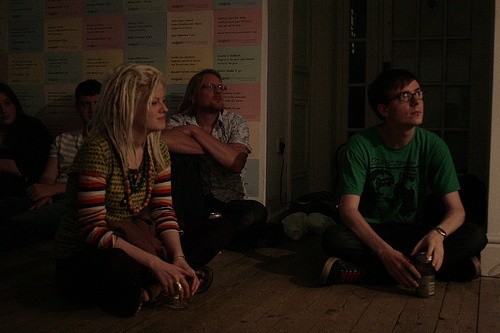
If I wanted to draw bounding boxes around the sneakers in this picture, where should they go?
[321,257,363,285]
[463,254,481,279]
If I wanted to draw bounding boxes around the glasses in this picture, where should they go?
[198,83,227,91]
[389,91,423,102]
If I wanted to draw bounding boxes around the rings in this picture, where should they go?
[174,281,183,291]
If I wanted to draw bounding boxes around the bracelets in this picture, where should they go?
[177,255,185,259]
[433,226,448,239]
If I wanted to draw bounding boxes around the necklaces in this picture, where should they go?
[103,125,154,212]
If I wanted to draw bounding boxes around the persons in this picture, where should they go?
[323,68,488,292]
[0,79,103,245]
[160,68,268,265]
[53,63,213,316]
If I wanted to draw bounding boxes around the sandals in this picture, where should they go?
[193,265,213,293]
[123,281,144,318]
